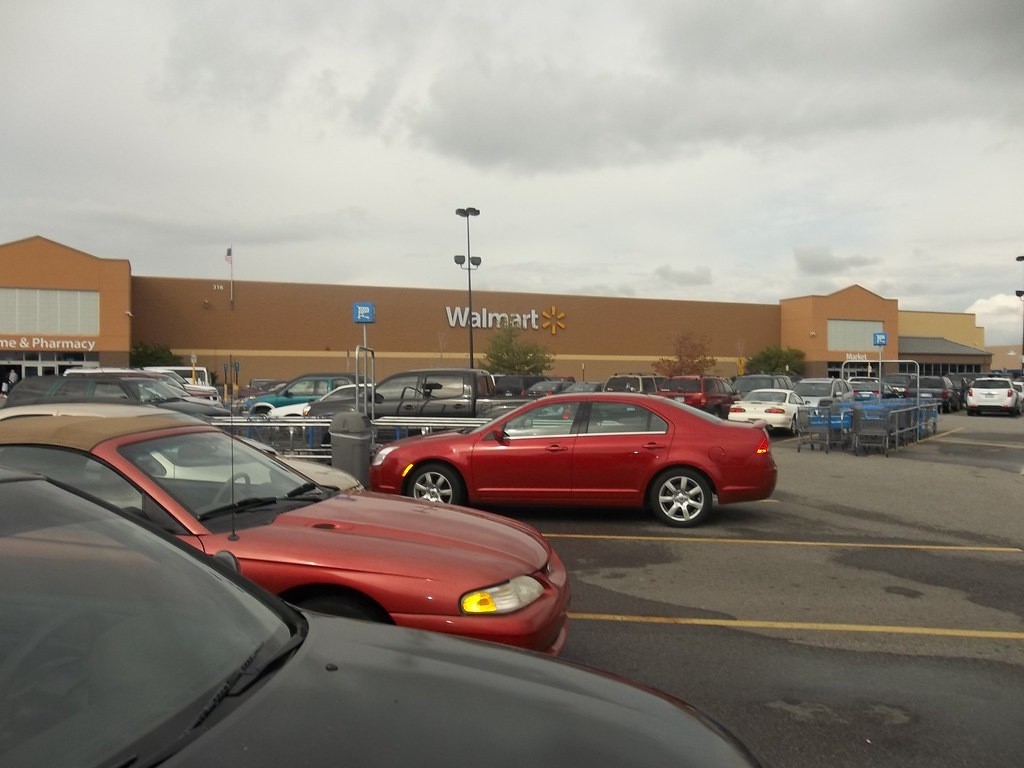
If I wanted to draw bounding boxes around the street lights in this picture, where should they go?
[1014,255,1024,382]
[454,208,481,367]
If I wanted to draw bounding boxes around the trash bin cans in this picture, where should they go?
[328,412,373,490]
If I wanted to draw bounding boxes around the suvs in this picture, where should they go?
[901,375,961,412]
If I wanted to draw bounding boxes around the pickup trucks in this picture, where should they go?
[304,369,581,463]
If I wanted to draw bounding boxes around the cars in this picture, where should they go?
[0,468,760,768]
[725,388,810,433]
[1010,382,1024,408]
[0,366,384,512]
[965,378,1022,416]
[489,371,921,420]
[0,403,574,664]
[367,391,777,528]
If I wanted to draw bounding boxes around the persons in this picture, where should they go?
[0,376,9,399]
[9,370,19,384]
